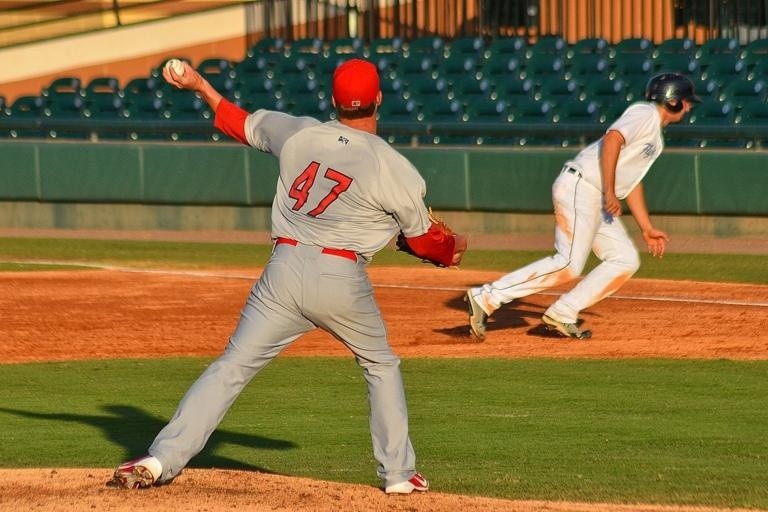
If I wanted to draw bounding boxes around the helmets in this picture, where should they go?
[646,72,702,112]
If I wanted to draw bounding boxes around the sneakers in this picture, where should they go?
[464,290,488,339]
[386,473,429,494]
[542,313,592,340]
[114,455,164,491]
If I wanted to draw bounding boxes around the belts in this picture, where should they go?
[563,166,582,178]
[275,238,357,260]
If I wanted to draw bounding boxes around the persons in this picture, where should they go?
[104,57,468,497]
[462,72,705,344]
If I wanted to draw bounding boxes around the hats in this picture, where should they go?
[333,60,380,109]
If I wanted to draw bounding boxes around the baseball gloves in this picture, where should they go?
[397,206,466,268]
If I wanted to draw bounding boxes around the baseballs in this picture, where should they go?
[164,59,186,78]
